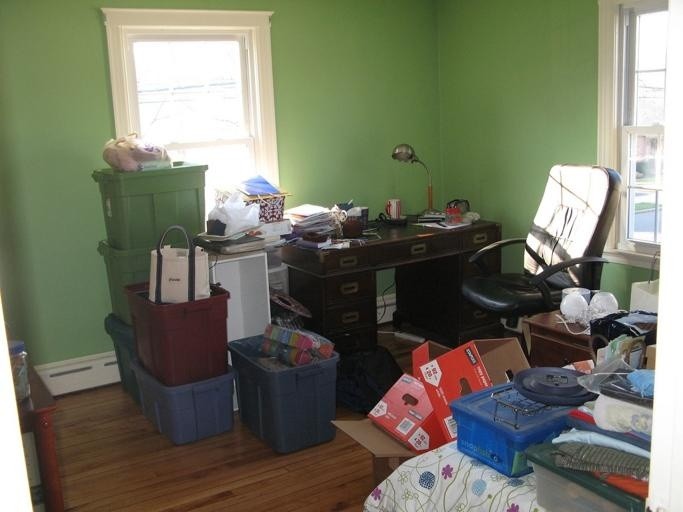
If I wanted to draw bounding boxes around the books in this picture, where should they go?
[193,203,351,255]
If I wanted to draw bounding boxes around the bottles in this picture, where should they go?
[7,342,31,403]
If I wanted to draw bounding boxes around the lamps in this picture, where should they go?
[393,144,444,218]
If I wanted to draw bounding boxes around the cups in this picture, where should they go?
[385,199,402,219]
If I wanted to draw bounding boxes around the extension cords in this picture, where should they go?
[395,331,425,344]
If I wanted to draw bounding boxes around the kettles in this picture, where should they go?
[445,199,471,225]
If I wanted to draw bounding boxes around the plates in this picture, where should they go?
[381,217,406,222]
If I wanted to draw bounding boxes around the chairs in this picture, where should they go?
[461,164,621,339]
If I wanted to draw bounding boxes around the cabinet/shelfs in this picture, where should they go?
[521,312,592,367]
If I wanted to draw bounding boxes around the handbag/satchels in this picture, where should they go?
[590,311,657,364]
[103,132,173,172]
[149,225,210,304]
[237,175,280,205]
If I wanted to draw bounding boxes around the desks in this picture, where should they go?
[19,357,64,512]
[279,218,503,408]
